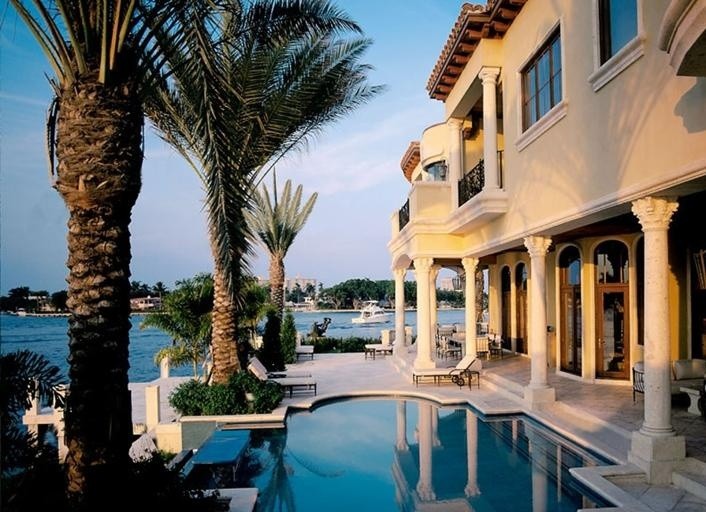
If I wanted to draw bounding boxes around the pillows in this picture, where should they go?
[693,357,705,380]
[673,358,696,384]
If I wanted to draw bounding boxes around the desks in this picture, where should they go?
[459,369,481,392]
[678,387,705,417]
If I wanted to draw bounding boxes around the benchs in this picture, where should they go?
[630,358,706,411]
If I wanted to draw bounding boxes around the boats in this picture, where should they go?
[349,296,391,325]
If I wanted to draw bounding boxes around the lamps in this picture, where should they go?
[436,157,448,179]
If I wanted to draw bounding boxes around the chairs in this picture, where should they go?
[411,353,478,389]
[244,342,316,399]
[362,320,506,364]
